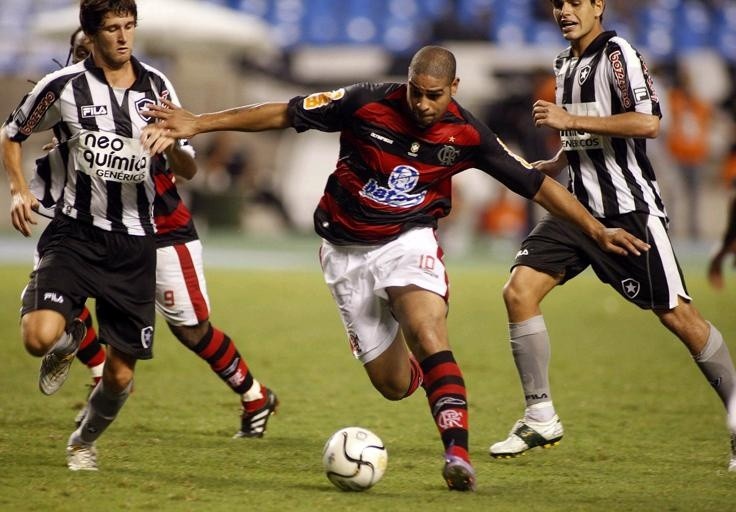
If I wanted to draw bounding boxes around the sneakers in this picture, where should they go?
[441,459,476,492]
[39,318,87,396]
[231,384,280,440]
[65,383,99,472]
[488,412,566,459]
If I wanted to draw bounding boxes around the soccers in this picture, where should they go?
[322,428,387,492]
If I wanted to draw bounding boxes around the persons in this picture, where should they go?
[23,25,281,444]
[140,45,656,490]
[0,0,198,470]
[488,1,736,470]
